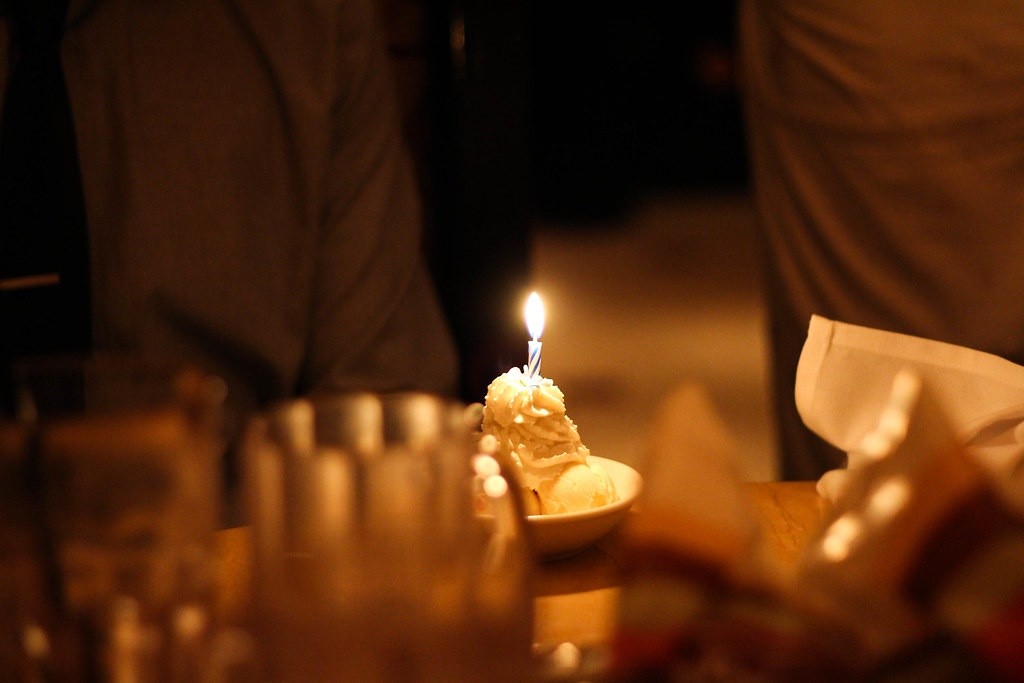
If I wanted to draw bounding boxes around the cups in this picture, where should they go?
[0,352,226,683]
[240,392,534,683]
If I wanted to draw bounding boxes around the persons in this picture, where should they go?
[0,0,463,502]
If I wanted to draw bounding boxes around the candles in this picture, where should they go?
[523,293,546,377]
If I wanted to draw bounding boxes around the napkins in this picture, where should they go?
[794,315,1024,521]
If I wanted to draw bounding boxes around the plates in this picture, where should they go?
[476,456,642,559]
